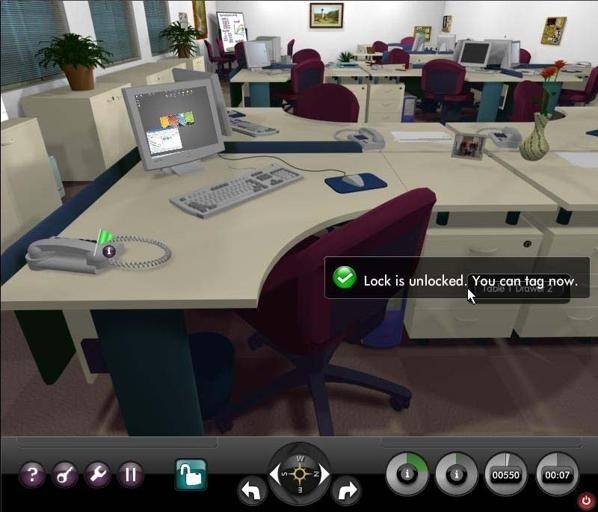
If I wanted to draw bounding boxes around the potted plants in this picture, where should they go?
[159,21,200,59]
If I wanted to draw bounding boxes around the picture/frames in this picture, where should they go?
[309,2,342,28]
